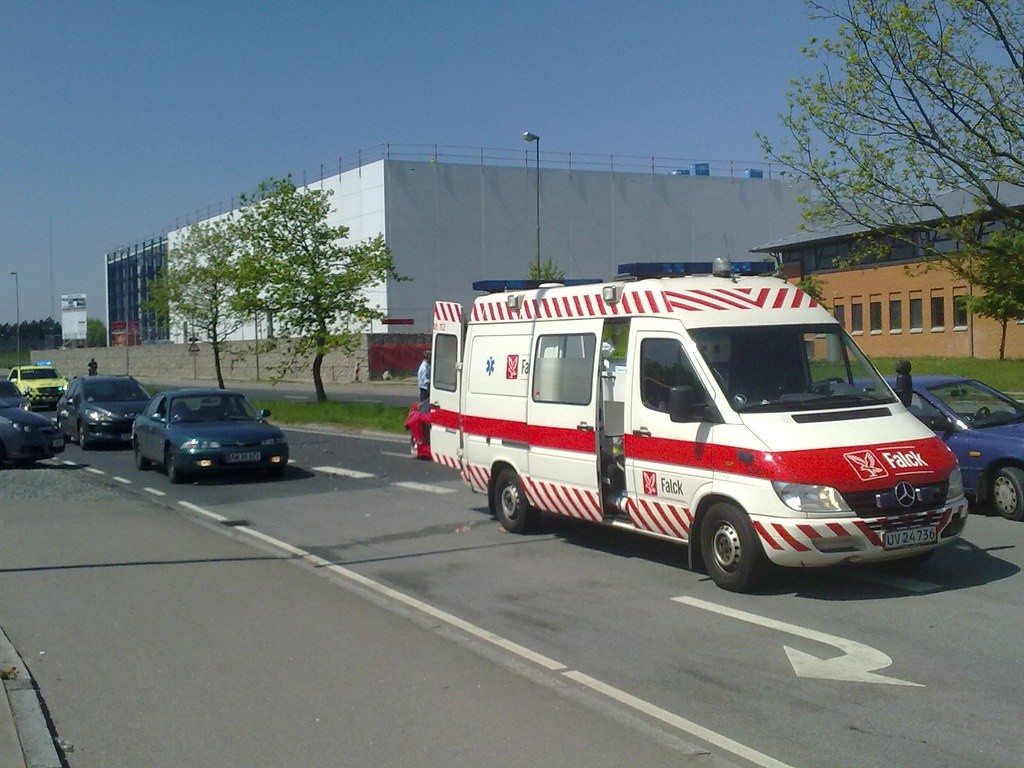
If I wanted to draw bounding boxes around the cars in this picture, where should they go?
[132,388,291,486]
[403,400,435,459]
[0,380,33,413]
[0,407,66,469]
[832,376,1024,525]
[7,365,71,410]
[56,375,158,453]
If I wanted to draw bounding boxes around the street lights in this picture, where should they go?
[523,131,545,291]
[9,272,21,366]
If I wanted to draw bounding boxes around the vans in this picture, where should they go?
[427,255,967,594]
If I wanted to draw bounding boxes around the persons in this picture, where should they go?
[215,395,241,420]
[87,357,99,376]
[416,348,431,402]
